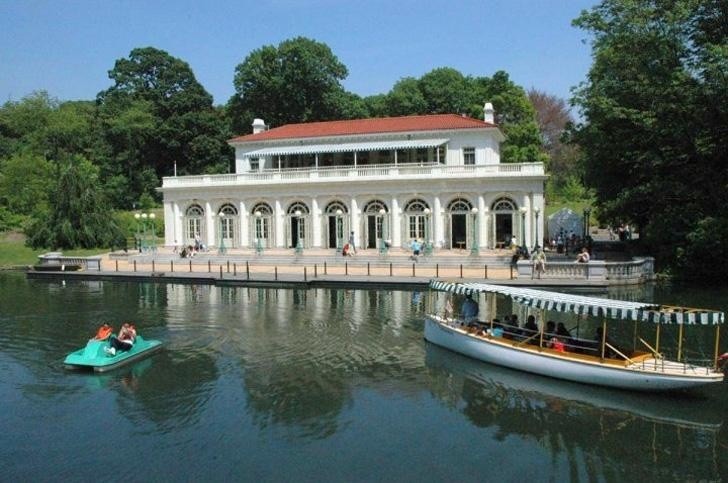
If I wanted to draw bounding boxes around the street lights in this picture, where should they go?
[254,210,264,257]
[218,212,227,255]
[470,208,479,258]
[134,213,158,256]
[294,210,303,256]
[518,206,528,254]
[335,209,344,257]
[422,207,433,257]
[582,207,591,236]
[531,206,540,255]
[378,208,388,257]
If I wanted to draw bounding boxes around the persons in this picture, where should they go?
[94,321,112,341]
[409,239,420,256]
[509,227,593,274]
[107,321,136,357]
[461,291,616,359]
[349,231,358,254]
[342,244,353,257]
[172,232,201,259]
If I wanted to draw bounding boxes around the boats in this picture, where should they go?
[62,333,163,372]
[423,278,727,390]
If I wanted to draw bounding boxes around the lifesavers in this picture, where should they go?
[717,353,728,368]
